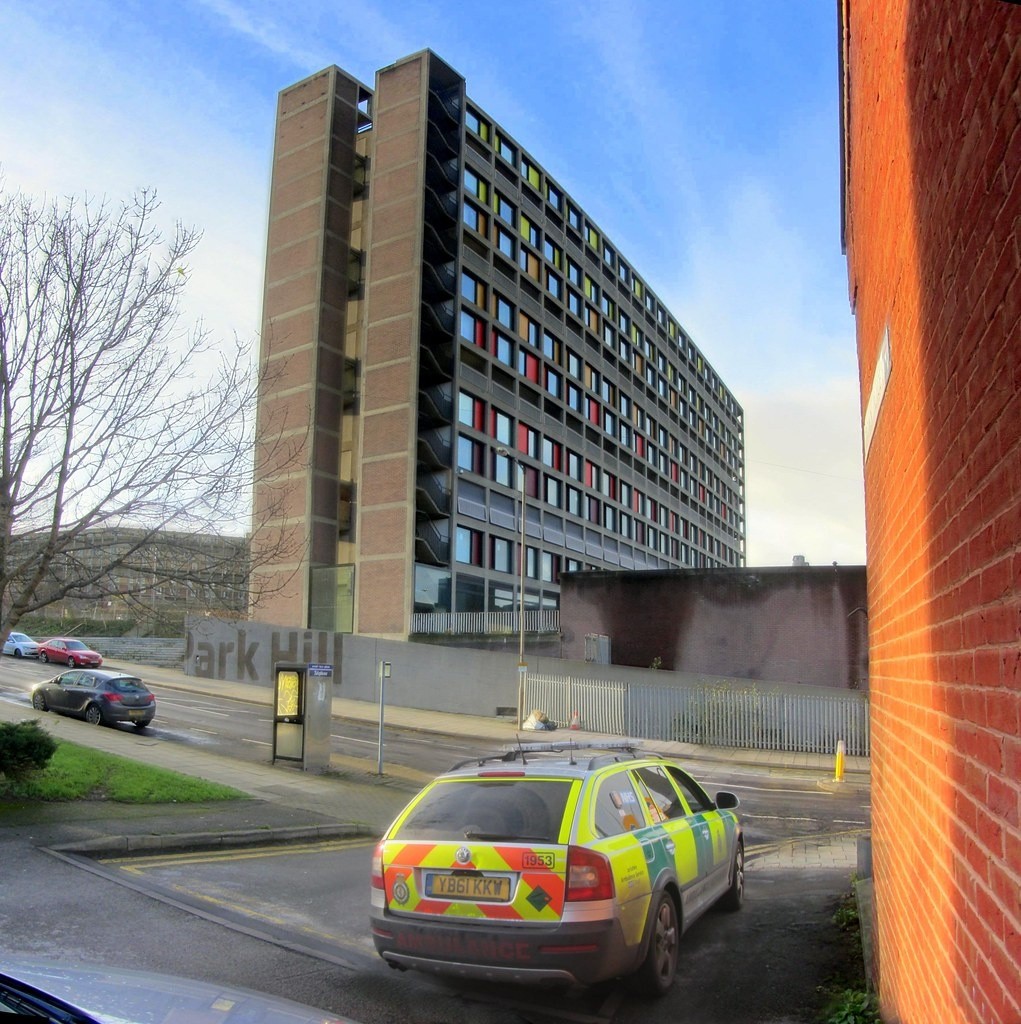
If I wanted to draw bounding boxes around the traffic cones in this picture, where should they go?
[571,710,581,731]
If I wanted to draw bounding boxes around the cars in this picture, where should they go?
[31,668,156,728]
[368,734,744,999]
[38,638,103,669]
[1,632,40,660]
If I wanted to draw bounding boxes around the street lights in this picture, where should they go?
[494,445,526,731]
